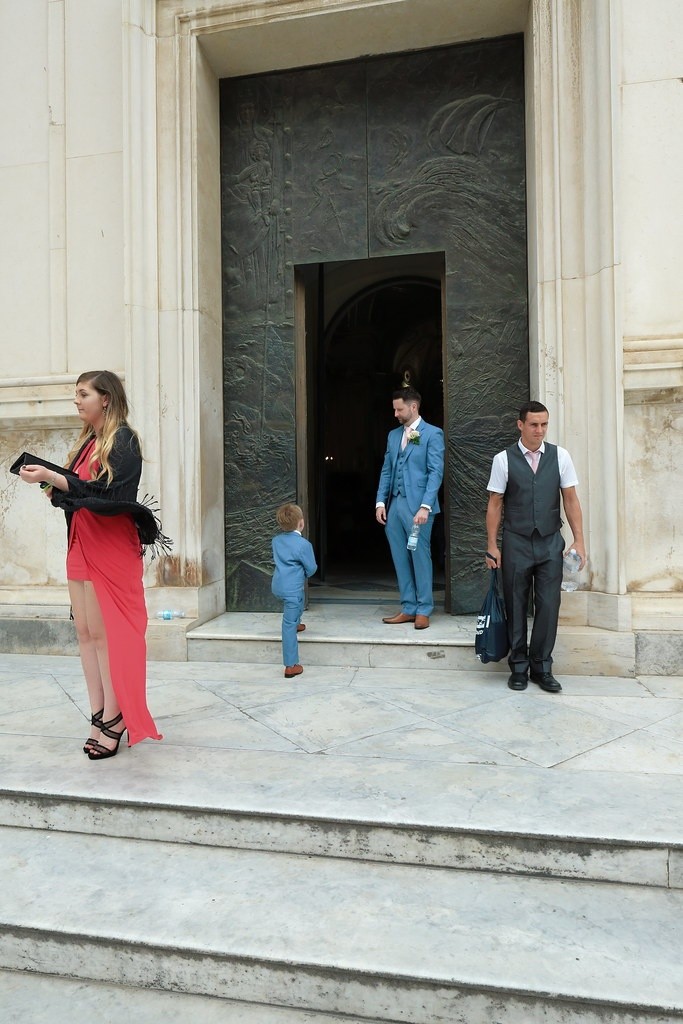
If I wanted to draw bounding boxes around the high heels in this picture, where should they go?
[88,711,131,760]
[84,707,105,754]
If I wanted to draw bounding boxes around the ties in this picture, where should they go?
[526,451,539,475]
[402,428,412,452]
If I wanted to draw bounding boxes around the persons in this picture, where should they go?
[486,400,586,691]
[18,370,174,761]
[271,504,318,678]
[375,388,445,629]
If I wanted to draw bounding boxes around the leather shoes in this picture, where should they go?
[414,615,430,629]
[297,624,306,632]
[507,672,530,690]
[529,668,562,691]
[284,664,304,678]
[382,611,416,625]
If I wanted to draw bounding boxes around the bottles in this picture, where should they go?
[155,610,185,620]
[561,549,582,593]
[406,522,420,551]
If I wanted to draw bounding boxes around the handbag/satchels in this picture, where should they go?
[474,551,511,664]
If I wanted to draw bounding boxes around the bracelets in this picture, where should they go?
[421,507,430,510]
[47,472,57,486]
